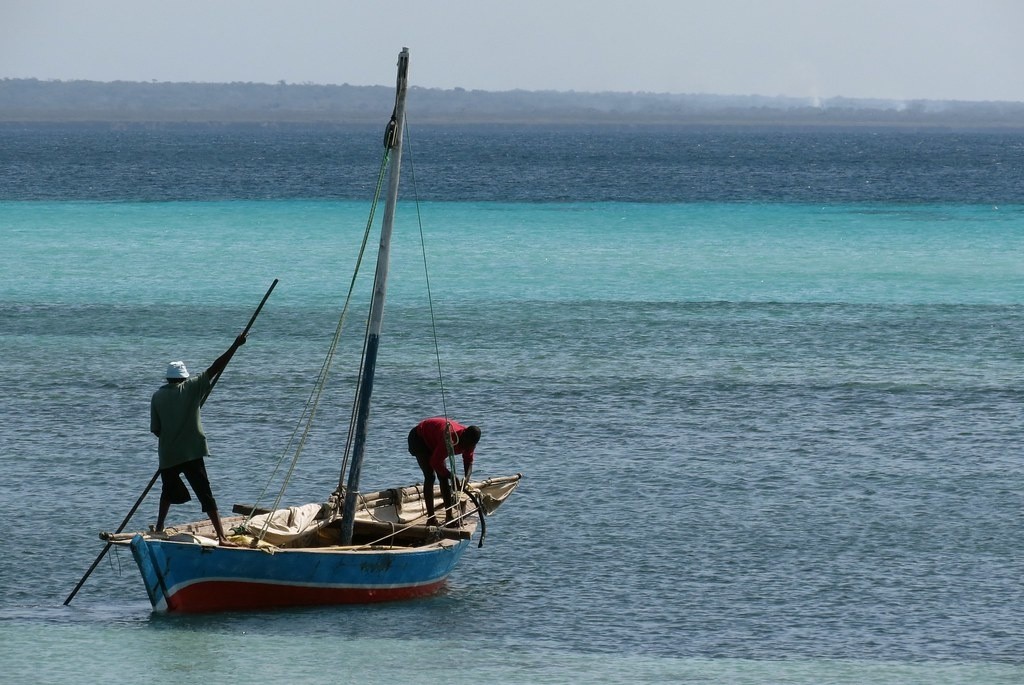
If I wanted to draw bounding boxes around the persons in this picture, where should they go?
[149,330,253,546]
[408,417,482,529]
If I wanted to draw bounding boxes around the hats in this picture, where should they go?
[165,360,189,378]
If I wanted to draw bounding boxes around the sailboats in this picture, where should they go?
[97,47,525,620]
